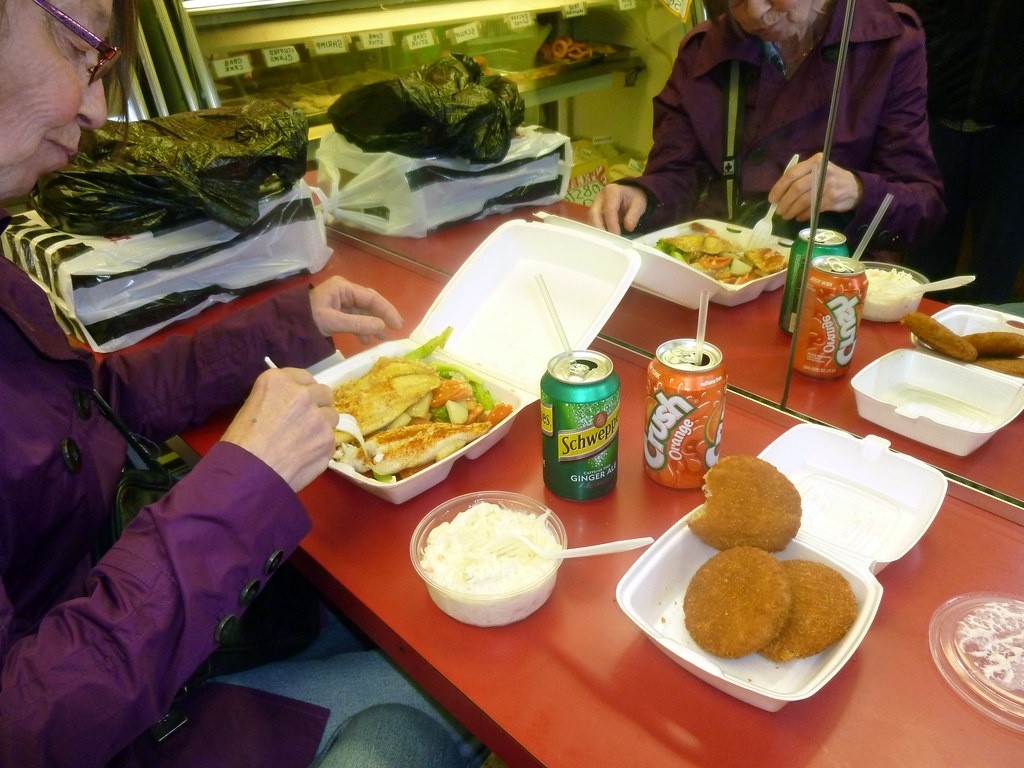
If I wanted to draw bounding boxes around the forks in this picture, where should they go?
[881,275,975,295]
[747,155,800,248]
[490,535,655,562]
[265,357,368,459]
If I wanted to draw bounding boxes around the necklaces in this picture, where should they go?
[782,34,824,69]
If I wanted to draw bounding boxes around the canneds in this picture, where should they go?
[642,338,727,490]
[540,349,620,501]
[791,256,869,380]
[778,227,850,335]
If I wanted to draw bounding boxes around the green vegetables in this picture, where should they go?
[401,324,493,423]
[653,239,690,263]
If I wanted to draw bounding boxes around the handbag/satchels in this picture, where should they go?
[112,463,318,679]
[0,179,331,353]
[328,52,527,165]
[26,95,310,236]
[308,117,573,239]
[732,196,812,240]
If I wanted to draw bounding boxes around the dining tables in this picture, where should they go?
[60,230,1023,768]
[294,169,1024,503]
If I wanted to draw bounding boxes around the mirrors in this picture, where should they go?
[122,1,1024,512]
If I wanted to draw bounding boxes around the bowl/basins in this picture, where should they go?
[410,491,568,627]
[860,261,929,322]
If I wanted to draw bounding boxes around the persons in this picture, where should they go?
[588,1,943,243]
[0,0,492,768]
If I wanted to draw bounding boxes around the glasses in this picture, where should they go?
[33,0,122,87]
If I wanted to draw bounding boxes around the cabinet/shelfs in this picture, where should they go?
[130,0,705,212]
